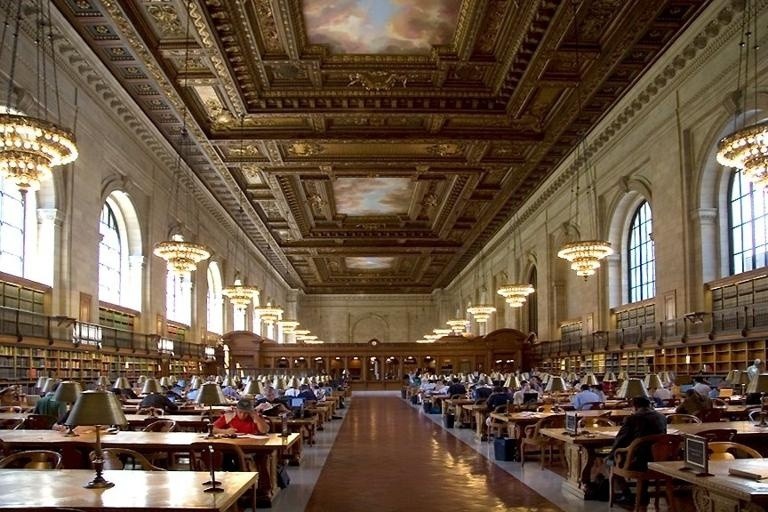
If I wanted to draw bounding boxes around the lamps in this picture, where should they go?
[254,170,285,328]
[417,370,768,427]
[294,254,323,345]
[219,114,259,315]
[277,225,300,334]
[557,3,613,280]
[152,1,210,274]
[715,3,767,189]
[0,0,80,199]
[465,176,497,324]
[497,113,535,309]
[35,372,333,489]
[413,210,471,344]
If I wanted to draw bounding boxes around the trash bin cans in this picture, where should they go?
[411,395,418,405]
[494,436,519,462]
[402,389,407,399]
[443,412,454,428]
[423,400,432,414]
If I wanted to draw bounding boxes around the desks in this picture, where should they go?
[0,384,350,512]
[400,383,768,512]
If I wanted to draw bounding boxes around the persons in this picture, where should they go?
[36,379,203,470]
[212,381,346,455]
[405,359,761,509]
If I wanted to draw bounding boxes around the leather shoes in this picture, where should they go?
[616,495,635,505]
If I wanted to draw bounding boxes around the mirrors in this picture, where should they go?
[275,356,475,382]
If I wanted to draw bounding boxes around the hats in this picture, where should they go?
[237,400,251,412]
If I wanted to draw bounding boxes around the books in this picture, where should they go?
[0,341,197,392]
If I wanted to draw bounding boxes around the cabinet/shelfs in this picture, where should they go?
[0,342,205,394]
[534,337,768,378]
